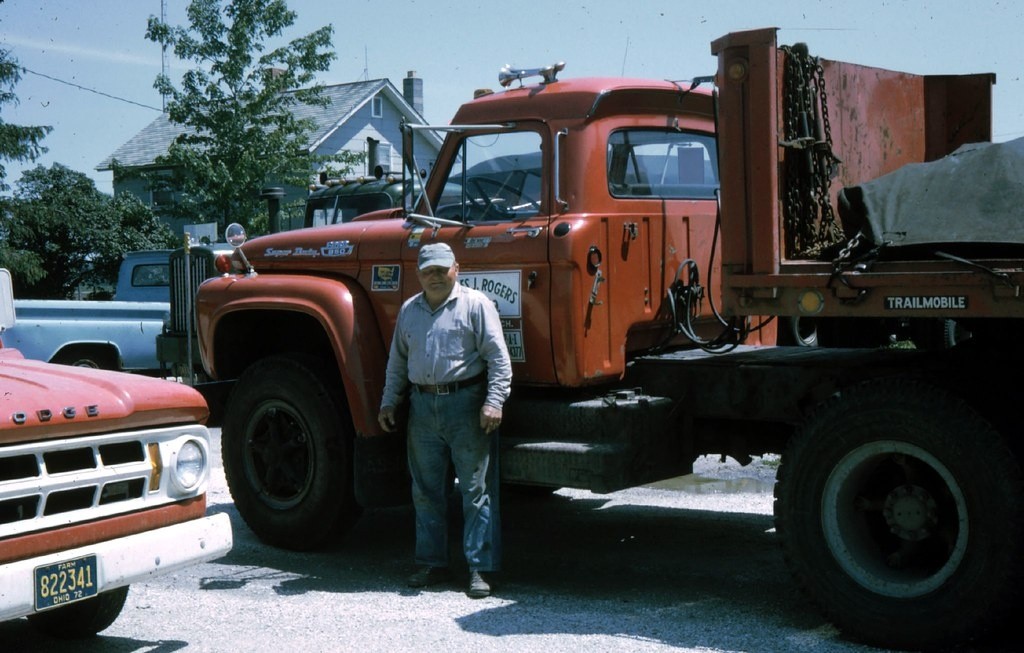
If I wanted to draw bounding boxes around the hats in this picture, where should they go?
[418,243,455,270]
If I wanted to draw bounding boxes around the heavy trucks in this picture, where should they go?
[195,29,1024,653]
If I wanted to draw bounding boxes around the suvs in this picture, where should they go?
[0,268,234,638]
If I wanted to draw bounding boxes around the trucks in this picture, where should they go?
[155,165,465,395]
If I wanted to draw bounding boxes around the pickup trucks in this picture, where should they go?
[1,250,180,377]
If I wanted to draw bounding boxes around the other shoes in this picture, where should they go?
[407,566,449,586]
[468,570,494,597]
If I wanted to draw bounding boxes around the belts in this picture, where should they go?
[411,373,484,396]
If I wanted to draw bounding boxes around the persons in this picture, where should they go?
[378,243,514,597]
